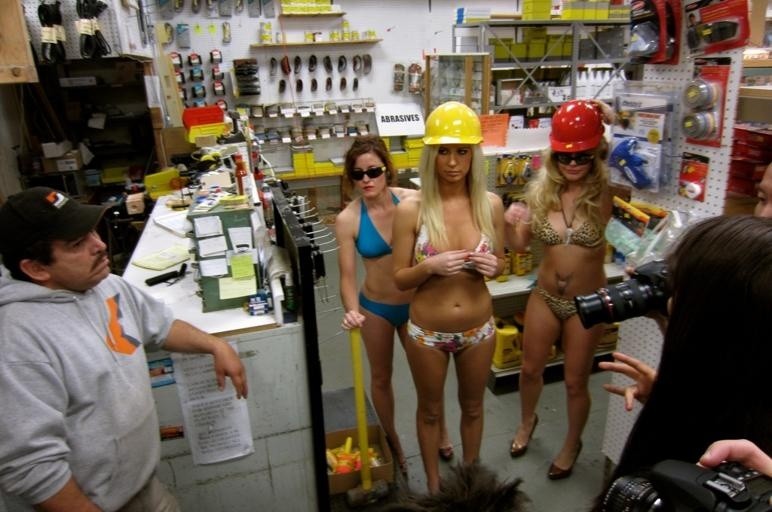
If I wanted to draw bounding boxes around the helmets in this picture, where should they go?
[422,101,484,145]
[550,99,605,153]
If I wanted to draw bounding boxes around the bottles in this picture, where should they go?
[306,19,376,42]
[114,192,127,205]
[235,159,273,221]
[563,69,626,100]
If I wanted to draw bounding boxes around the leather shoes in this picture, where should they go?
[549,440,582,479]
[511,413,538,456]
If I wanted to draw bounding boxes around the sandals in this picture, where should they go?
[438,441,454,460]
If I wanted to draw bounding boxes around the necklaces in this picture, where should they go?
[557,191,581,246]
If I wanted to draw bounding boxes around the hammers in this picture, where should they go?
[346,317,389,508]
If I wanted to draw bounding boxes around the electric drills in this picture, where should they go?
[606,137,653,190]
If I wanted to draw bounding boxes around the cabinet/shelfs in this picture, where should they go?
[0,0,169,206]
[482,128,625,396]
[452,20,633,127]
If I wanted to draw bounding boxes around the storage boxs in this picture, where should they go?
[325,424,398,494]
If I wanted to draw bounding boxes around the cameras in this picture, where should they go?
[574,259,676,330]
[601,459,772,512]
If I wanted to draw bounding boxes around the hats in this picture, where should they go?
[0,186,109,254]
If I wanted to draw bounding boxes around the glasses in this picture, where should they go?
[555,152,596,165]
[350,165,387,180]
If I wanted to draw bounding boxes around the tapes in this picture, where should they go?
[680,80,719,141]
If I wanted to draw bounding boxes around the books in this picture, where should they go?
[151,209,193,236]
[132,246,190,271]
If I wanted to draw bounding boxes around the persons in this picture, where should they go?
[696,438,771,478]
[0,188,248,512]
[502,93,620,481]
[335,456,534,511]
[586,214,770,512]
[335,133,454,479]
[598,160,772,412]
[392,101,506,497]
